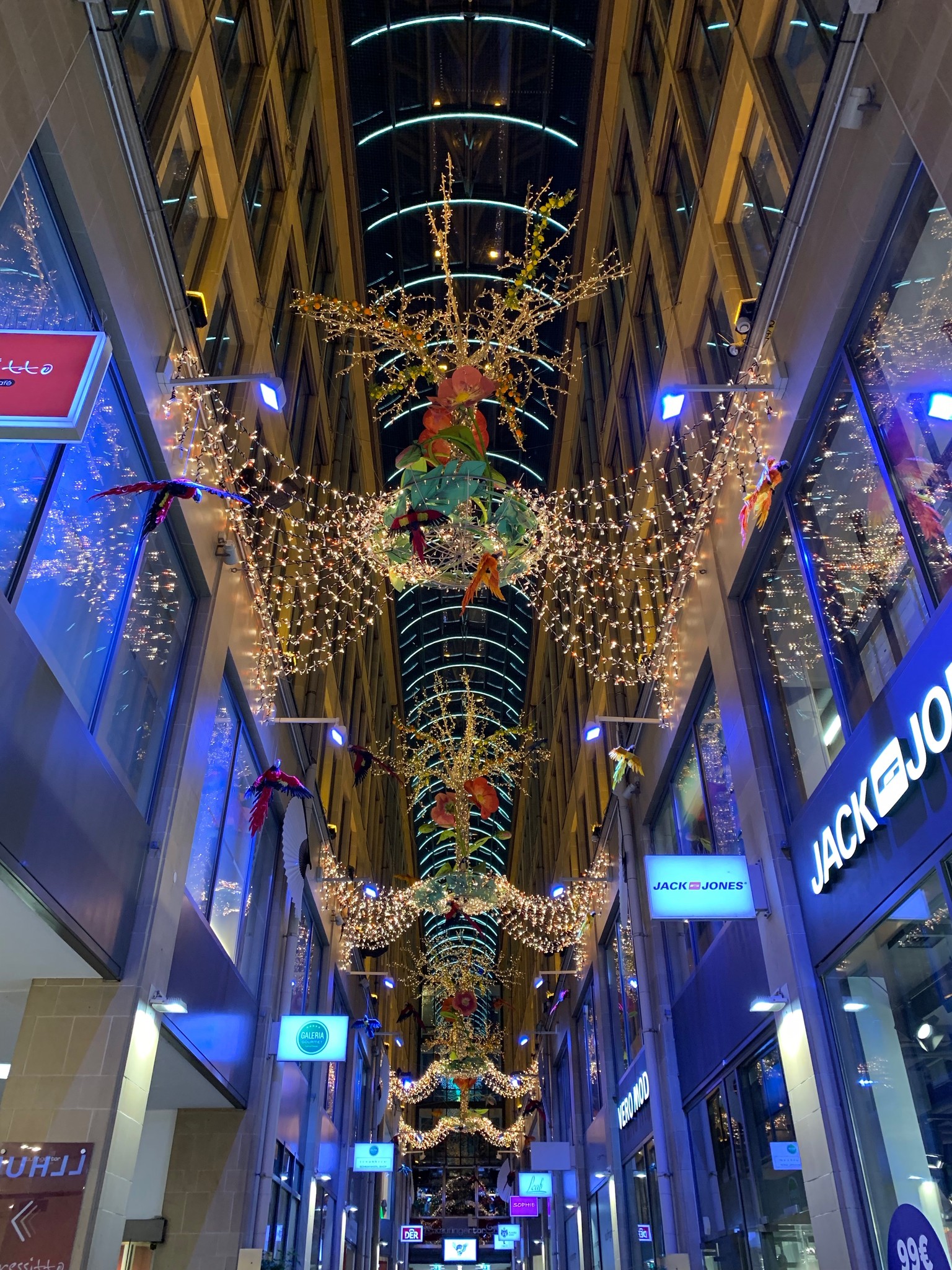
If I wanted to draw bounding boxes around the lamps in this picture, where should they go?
[373,1032,405,1048]
[659,360,790,420]
[519,1023,558,1046]
[315,866,378,898]
[534,971,577,989]
[156,355,287,414]
[551,866,613,898]
[345,962,395,984]
[584,703,669,741]
[266,702,348,747]
[509,1072,528,1088]
